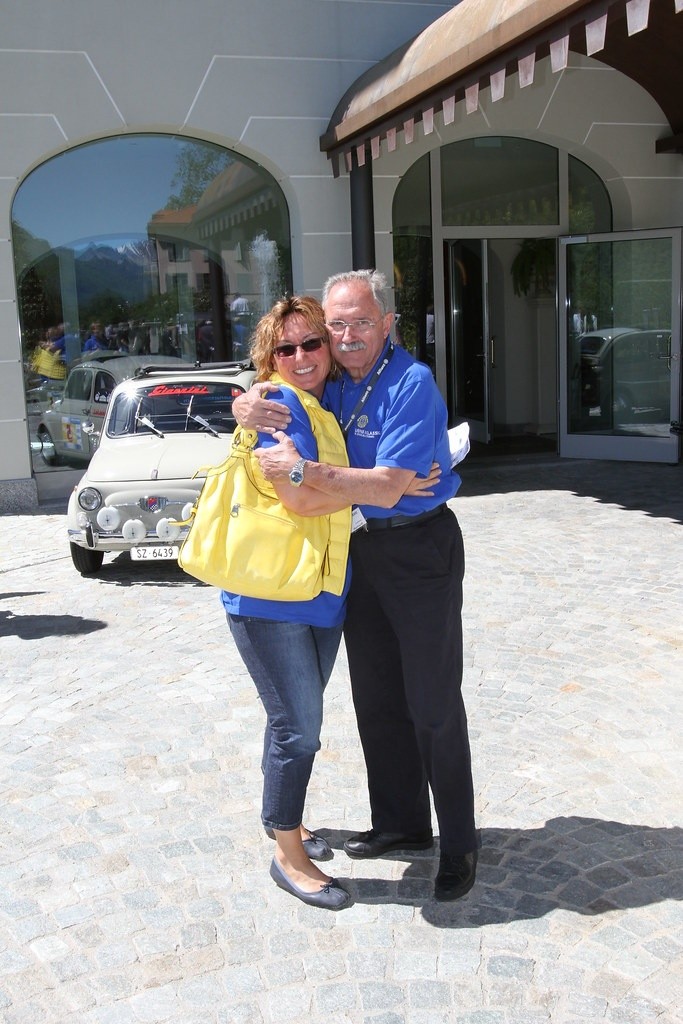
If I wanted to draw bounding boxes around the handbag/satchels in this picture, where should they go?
[176,388,329,602]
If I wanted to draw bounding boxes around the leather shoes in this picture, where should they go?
[342,828,435,860]
[433,849,479,901]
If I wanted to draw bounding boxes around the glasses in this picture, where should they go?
[323,315,386,336]
[272,335,330,358]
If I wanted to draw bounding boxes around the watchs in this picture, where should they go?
[289,458,308,487]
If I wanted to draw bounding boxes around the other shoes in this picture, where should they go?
[269,856,351,909]
[263,822,331,860]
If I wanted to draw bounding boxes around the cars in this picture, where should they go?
[576,326,673,424]
[67,358,257,574]
[37,355,188,467]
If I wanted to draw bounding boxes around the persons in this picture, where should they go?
[426,305,436,354]
[38,292,252,409]
[215,295,442,909]
[232,268,478,901]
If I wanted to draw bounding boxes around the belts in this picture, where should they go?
[353,501,448,532]
